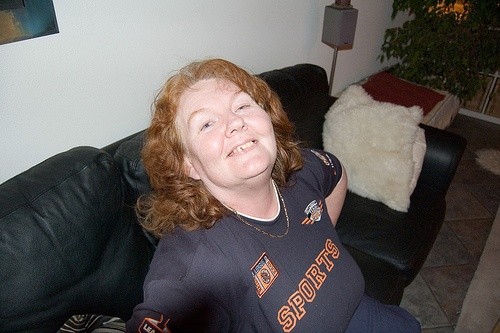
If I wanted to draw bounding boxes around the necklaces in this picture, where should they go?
[223,179,290,238]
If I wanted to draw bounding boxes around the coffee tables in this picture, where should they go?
[355,71,461,130]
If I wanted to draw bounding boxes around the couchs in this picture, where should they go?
[0,64,468,333]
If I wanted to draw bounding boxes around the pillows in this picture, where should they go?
[322,83,426,212]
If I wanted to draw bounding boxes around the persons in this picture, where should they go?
[125,58,422,333]
